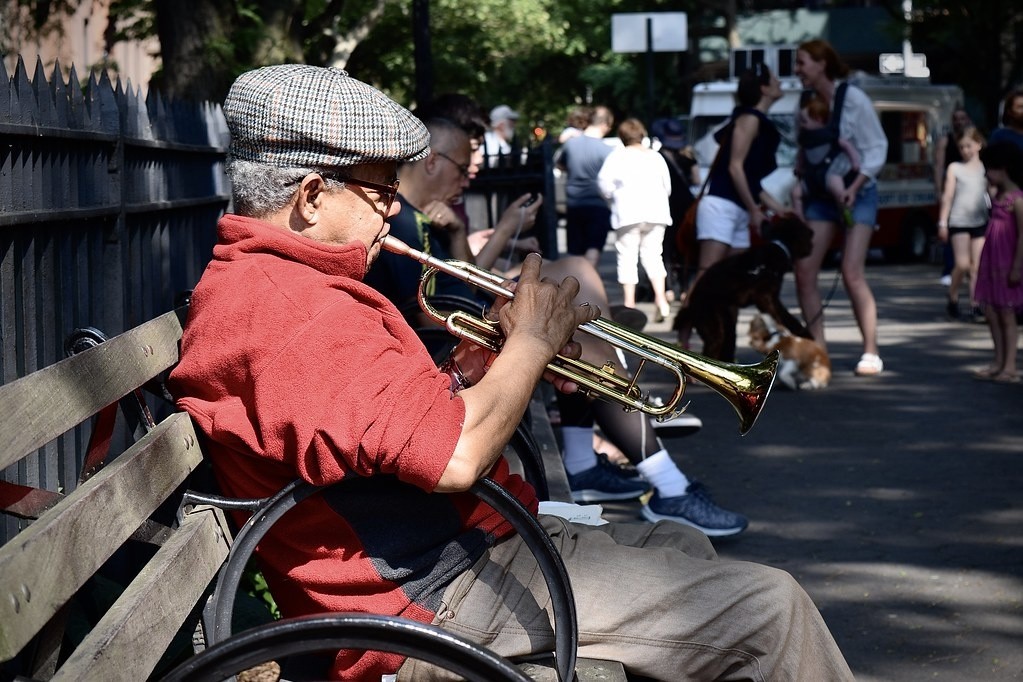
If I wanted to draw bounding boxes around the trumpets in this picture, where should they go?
[379,234,780,438]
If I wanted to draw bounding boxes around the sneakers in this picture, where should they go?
[640,481,748,536]
[563,451,651,501]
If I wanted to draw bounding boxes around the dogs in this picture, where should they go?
[749,314,831,391]
[673,213,816,359]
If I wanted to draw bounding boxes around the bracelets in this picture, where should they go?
[938,224,948,229]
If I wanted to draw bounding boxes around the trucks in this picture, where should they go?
[860,84,964,263]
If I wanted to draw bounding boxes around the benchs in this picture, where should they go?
[0,299,629,682]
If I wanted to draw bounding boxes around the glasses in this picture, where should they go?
[283,170,400,210]
[438,153,469,178]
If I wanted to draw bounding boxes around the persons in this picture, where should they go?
[936,90,1023,386]
[384,39,891,535]
[164,64,856,682]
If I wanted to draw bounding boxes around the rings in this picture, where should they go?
[436,213,442,219]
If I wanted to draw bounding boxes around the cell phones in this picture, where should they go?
[524,194,538,207]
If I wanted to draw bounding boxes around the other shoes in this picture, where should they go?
[596,438,636,470]
[677,343,698,384]
[970,306,986,322]
[972,368,1020,384]
[799,378,827,390]
[855,354,883,376]
[640,394,702,438]
[655,304,670,322]
[946,302,961,321]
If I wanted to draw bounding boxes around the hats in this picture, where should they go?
[223,64,431,169]
[489,105,520,120]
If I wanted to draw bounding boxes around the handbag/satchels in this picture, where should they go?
[676,198,702,272]
